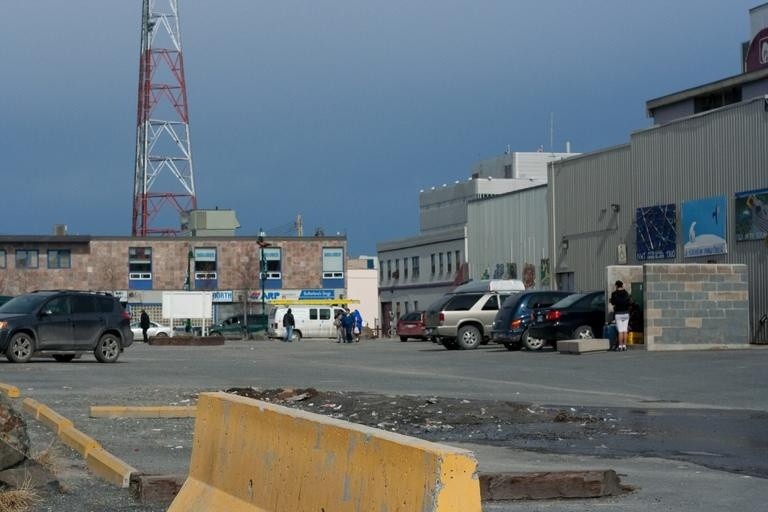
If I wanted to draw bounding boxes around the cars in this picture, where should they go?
[395,279,605,352]
[131,322,174,344]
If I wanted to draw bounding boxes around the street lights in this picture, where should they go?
[256,227,266,331]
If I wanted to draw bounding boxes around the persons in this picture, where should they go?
[351,309,363,343]
[282,308,295,342]
[333,310,345,344]
[342,309,354,343]
[610,280,631,351]
[185,319,191,334]
[139,308,150,344]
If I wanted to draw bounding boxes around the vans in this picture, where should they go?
[0,289,133,364]
[198,314,267,340]
[267,302,347,341]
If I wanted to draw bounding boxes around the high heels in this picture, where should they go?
[619,344,627,352]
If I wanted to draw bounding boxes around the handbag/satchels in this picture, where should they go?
[334,319,341,327]
[353,327,360,334]
